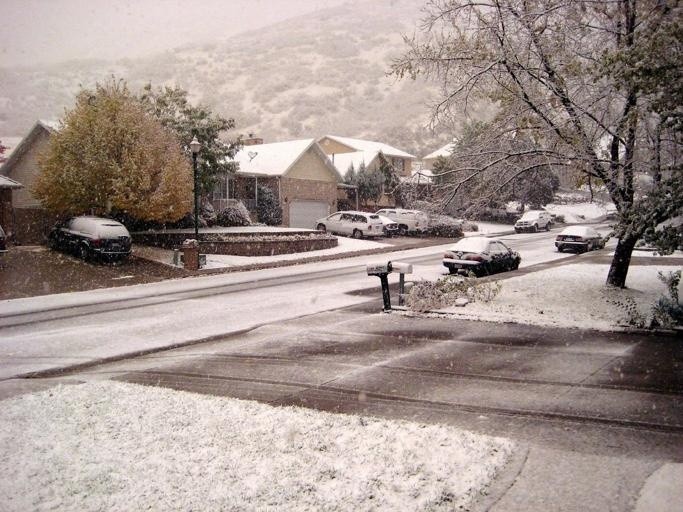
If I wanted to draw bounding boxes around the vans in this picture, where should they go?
[375,209,429,236]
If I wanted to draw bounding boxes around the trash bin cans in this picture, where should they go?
[178,241,200,272]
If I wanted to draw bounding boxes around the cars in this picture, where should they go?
[443,237,521,273]
[0,226,8,257]
[315,210,398,239]
[48,217,132,263]
[555,226,605,253]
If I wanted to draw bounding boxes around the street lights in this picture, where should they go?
[190,135,201,268]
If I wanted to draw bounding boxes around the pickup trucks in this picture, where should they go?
[515,211,555,233]
